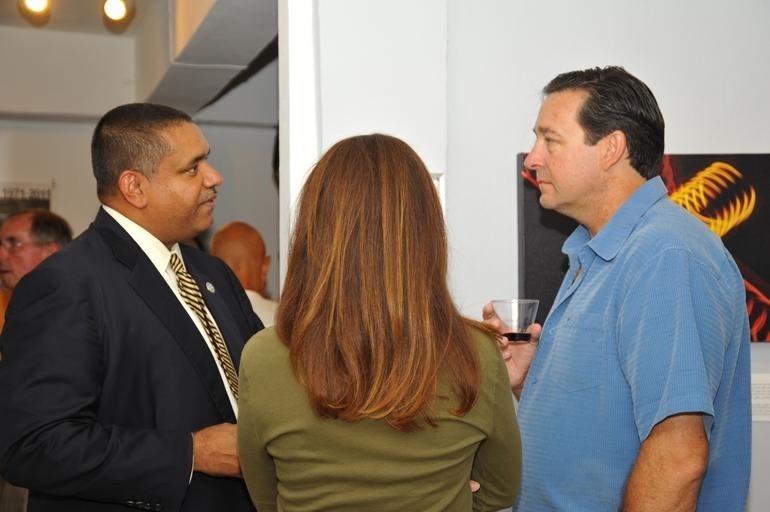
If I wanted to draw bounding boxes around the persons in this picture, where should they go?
[1,209,74,291]
[235,133,526,512]
[209,219,283,331]
[480,65,754,512]
[2,101,267,512]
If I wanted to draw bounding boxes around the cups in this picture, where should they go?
[491,300,540,344]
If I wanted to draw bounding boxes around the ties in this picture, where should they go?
[171,253,239,403]
